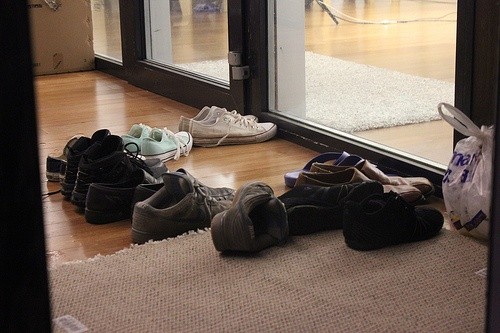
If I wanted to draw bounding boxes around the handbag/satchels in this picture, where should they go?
[438,103,494,241]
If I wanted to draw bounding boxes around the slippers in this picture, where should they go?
[283,151,377,185]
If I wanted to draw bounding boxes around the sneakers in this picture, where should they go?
[61,128,169,207]
[211,181,288,255]
[179,106,278,145]
[45,134,146,184]
[121,122,193,161]
[130,168,235,244]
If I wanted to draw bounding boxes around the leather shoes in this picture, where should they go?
[85,168,160,224]
[131,168,235,221]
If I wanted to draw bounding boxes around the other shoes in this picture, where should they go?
[341,190,444,250]
[293,159,433,201]
[276,181,384,235]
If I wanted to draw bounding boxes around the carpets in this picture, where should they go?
[172,51,455,133]
[48,228,488,333]
